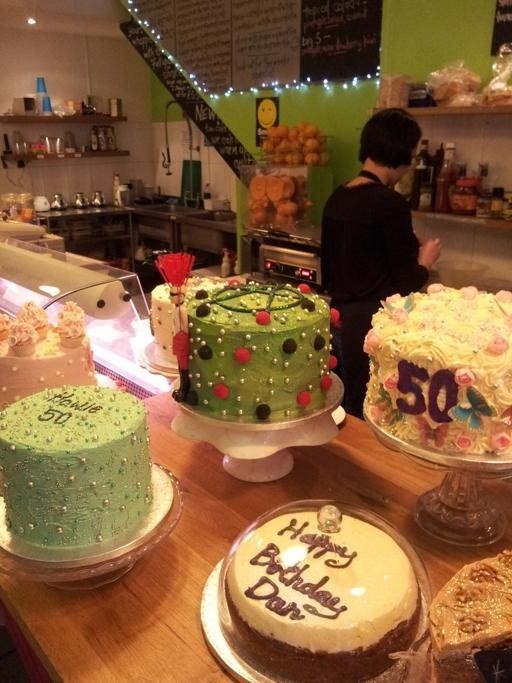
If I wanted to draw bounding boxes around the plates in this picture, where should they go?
[199,546,432,683]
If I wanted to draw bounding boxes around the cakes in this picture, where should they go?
[0,385,153,551]
[427,549,512,683]
[0,298,99,414]
[363,283,512,462]
[185,279,339,424]
[151,275,227,365]
[225,510,423,683]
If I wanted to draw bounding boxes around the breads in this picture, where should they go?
[248,173,314,232]
[379,63,512,110]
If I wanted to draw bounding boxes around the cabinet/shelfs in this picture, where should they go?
[1,112,130,162]
[35,204,134,278]
[409,102,512,229]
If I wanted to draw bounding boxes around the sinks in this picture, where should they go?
[136,204,201,243]
[181,211,236,254]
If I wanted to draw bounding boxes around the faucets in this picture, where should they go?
[161,99,194,200]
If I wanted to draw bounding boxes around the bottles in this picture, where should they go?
[50,187,106,210]
[203,182,211,209]
[90,124,118,151]
[490,186,506,219]
[11,129,24,156]
[111,172,131,208]
[411,138,457,215]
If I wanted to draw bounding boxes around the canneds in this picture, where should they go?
[448,179,478,215]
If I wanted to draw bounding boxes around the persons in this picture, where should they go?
[319,104,443,424]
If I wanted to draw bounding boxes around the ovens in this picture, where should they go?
[256,243,321,287]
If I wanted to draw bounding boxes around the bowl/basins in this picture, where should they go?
[433,260,490,289]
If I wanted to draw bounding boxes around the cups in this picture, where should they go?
[54,136,65,154]
[44,136,55,154]
[36,76,47,95]
[41,95,54,116]
